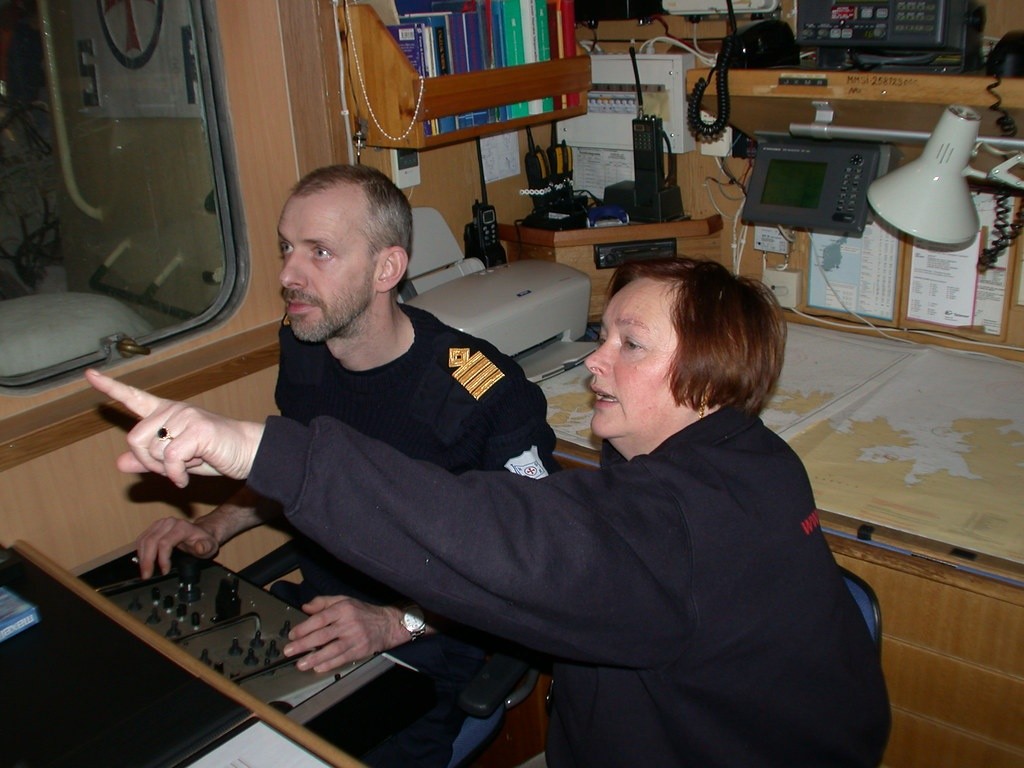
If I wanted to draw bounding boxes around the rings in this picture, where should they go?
[156,427,175,441]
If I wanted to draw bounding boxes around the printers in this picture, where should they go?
[395,206,602,383]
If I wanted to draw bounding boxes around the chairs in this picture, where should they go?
[237,542,543,768]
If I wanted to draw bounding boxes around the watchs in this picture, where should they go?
[399,613,426,641]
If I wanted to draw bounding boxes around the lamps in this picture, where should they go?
[865,104,1024,246]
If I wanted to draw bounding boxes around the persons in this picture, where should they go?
[137,163,566,768]
[83,251,895,768]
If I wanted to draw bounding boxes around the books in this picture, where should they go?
[0,587,40,642]
[385,0,581,136]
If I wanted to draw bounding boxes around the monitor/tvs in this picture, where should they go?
[761,158,827,210]
